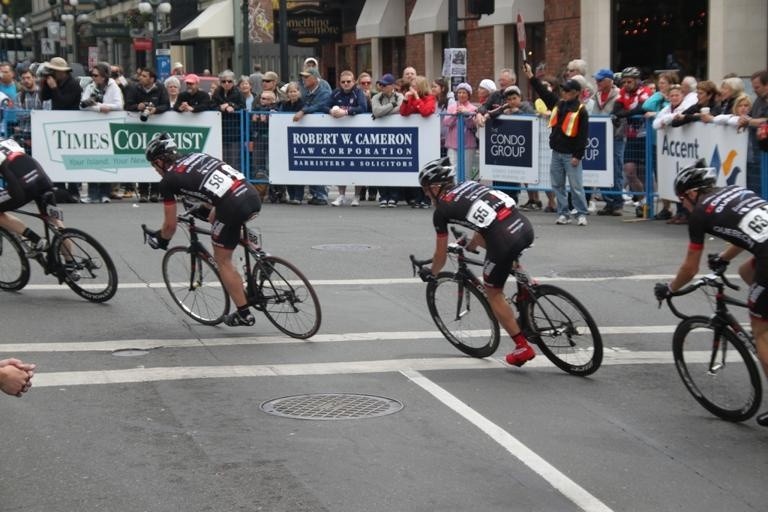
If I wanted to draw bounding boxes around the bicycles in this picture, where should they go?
[142,197,322,339]
[410,227,603,376]
[657,267,763,422]
[0,190,118,302]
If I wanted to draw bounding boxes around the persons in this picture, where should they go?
[357,73,378,113]
[164,76,180,110]
[654,163,768,425]
[593,73,656,207]
[1,358,36,398]
[535,77,559,213]
[173,74,210,113]
[567,60,587,78]
[251,71,288,110]
[478,79,498,102]
[672,81,716,127]
[568,75,594,215]
[400,76,431,209]
[236,75,257,110]
[323,71,366,207]
[523,63,591,226]
[125,68,170,114]
[738,69,768,195]
[145,131,262,327]
[293,68,330,121]
[38,56,83,203]
[371,74,403,208]
[170,63,184,76]
[591,69,625,216]
[484,85,542,212]
[433,78,455,166]
[253,90,276,122]
[304,58,332,96]
[0,136,81,285]
[323,71,367,118]
[210,80,219,92]
[475,68,521,206]
[249,64,265,96]
[443,82,478,184]
[0,61,23,136]
[613,67,653,218]
[283,82,305,112]
[652,85,691,224]
[79,64,124,204]
[211,70,246,113]
[714,95,751,126]
[111,65,143,88]
[700,78,744,124]
[15,69,52,137]
[29,61,52,82]
[403,67,417,87]
[416,157,538,366]
[642,72,679,220]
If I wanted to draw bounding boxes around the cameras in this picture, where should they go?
[37,70,53,78]
[81,88,103,108]
[141,97,157,122]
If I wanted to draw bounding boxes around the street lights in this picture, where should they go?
[138,2,172,78]
[0,14,13,59]
[15,17,31,57]
[61,1,88,62]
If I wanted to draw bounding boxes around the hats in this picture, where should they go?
[456,82,474,97]
[562,79,582,92]
[592,70,614,80]
[479,79,498,94]
[299,68,319,77]
[304,57,318,68]
[45,57,73,71]
[377,74,396,85]
[184,74,200,84]
[504,86,522,97]
[260,73,278,81]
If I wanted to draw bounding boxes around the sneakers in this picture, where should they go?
[597,205,611,217]
[25,239,51,257]
[351,198,361,207]
[259,254,276,279]
[331,197,345,206]
[388,199,398,208]
[612,206,625,216]
[122,191,134,198]
[79,195,100,204]
[223,312,255,327]
[654,208,672,219]
[149,194,160,202]
[506,344,536,365]
[666,213,689,225]
[380,199,388,208]
[61,266,82,281]
[577,213,589,226]
[556,215,573,224]
[520,200,543,211]
[139,194,148,203]
[101,195,112,203]
[517,281,538,303]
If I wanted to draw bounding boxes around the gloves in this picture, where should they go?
[708,253,730,270]
[654,281,672,300]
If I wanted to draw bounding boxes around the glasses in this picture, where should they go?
[669,83,682,90]
[378,83,390,88]
[222,80,234,84]
[149,158,162,166]
[262,78,274,83]
[361,80,372,86]
[341,80,354,84]
[422,184,434,192]
[91,73,103,77]
[679,194,690,202]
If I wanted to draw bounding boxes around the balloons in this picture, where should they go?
[535,98,547,116]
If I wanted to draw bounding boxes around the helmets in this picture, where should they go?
[419,157,456,186]
[622,67,641,80]
[145,135,178,160]
[674,166,718,196]
